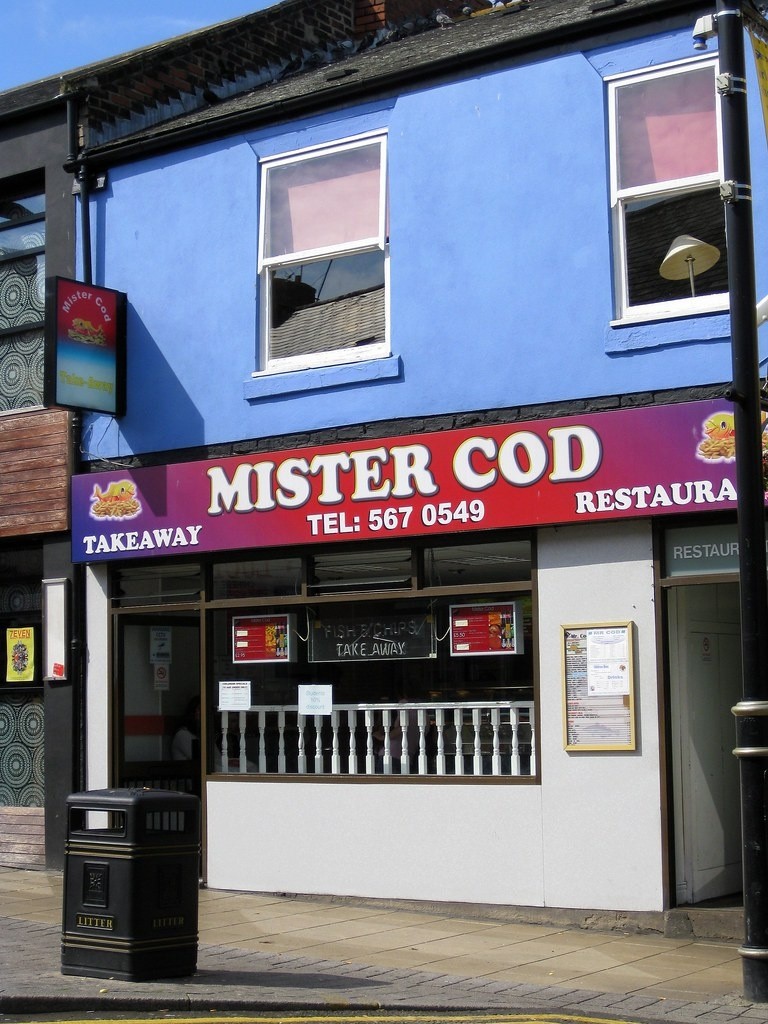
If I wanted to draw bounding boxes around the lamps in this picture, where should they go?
[660,236,721,298]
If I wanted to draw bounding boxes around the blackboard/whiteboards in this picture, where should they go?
[308,614,437,662]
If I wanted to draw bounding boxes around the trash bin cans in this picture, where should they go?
[60,787,203,982]
